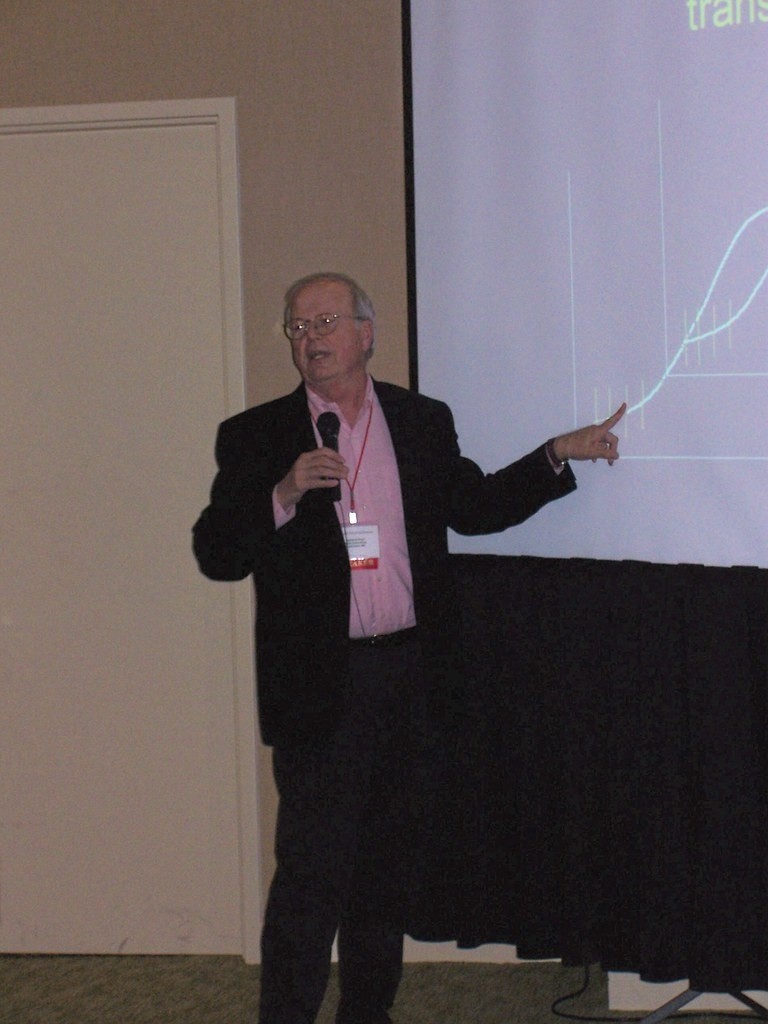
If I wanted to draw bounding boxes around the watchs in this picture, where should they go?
[547,437,570,467]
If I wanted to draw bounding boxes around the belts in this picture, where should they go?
[349,626,419,649]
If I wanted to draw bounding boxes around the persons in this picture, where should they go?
[192,272,626,1024]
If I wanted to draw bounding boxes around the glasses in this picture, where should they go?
[283,313,372,340]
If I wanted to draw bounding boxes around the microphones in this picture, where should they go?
[316,411,341,500]
